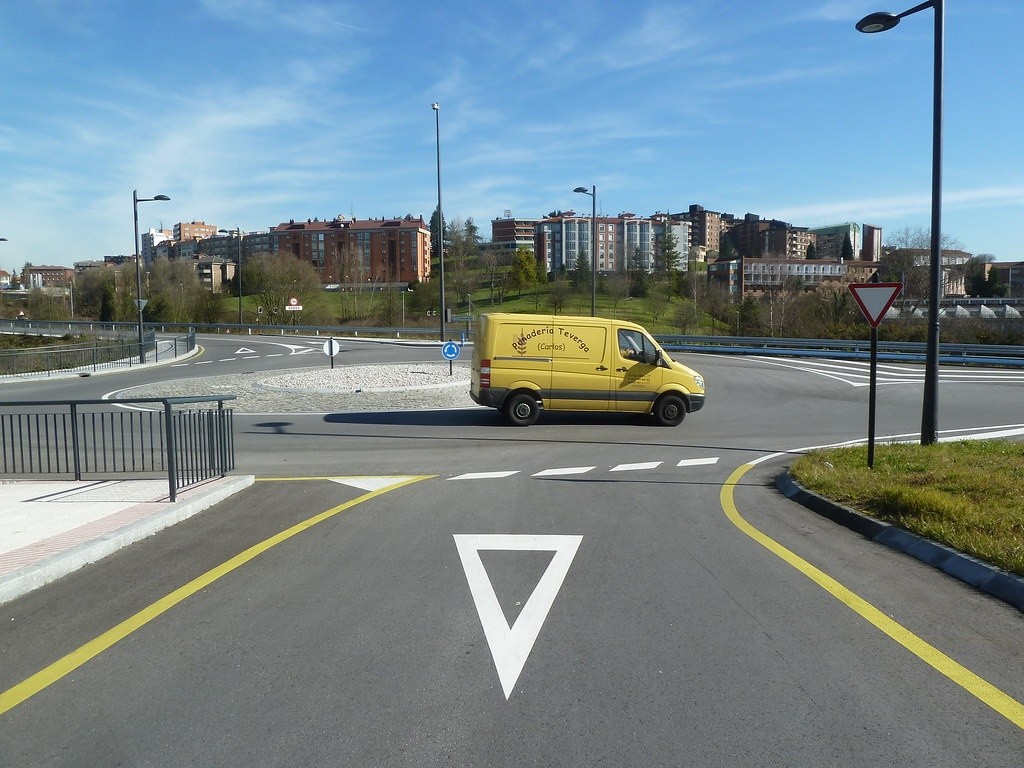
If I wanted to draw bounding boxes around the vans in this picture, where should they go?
[469,312,704,426]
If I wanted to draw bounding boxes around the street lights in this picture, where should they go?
[218,227,242,333]
[855,0,944,447]
[430,101,446,344]
[132,189,172,364]
[572,184,597,317]
